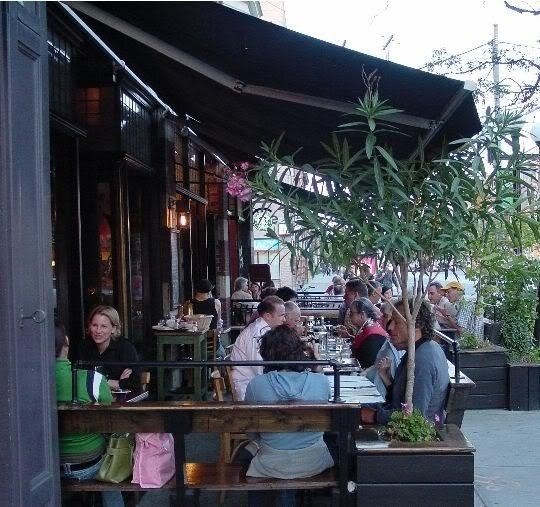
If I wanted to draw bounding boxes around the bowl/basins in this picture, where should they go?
[184,314,214,330]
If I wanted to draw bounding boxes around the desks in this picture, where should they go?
[154,327,207,401]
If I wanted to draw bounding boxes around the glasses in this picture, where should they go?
[349,311,362,315]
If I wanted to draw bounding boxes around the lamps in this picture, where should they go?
[169,198,191,229]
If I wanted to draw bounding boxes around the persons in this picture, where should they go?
[189,277,228,374]
[440,279,485,349]
[227,294,287,401]
[324,296,452,457]
[348,295,402,399]
[77,303,144,402]
[55,319,127,507]
[230,259,400,341]
[427,281,458,344]
[243,321,337,506]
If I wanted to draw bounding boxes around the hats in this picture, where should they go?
[196,279,215,291]
[441,281,465,290]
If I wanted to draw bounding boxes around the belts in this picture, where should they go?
[60,448,107,471]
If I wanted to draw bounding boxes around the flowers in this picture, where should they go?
[388,402,442,441]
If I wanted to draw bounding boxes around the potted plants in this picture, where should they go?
[465,238,514,346]
[449,330,508,408]
[500,260,540,411]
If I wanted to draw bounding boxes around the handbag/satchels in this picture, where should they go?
[95,432,176,489]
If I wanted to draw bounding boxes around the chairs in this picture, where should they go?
[211,370,250,462]
[207,329,217,387]
